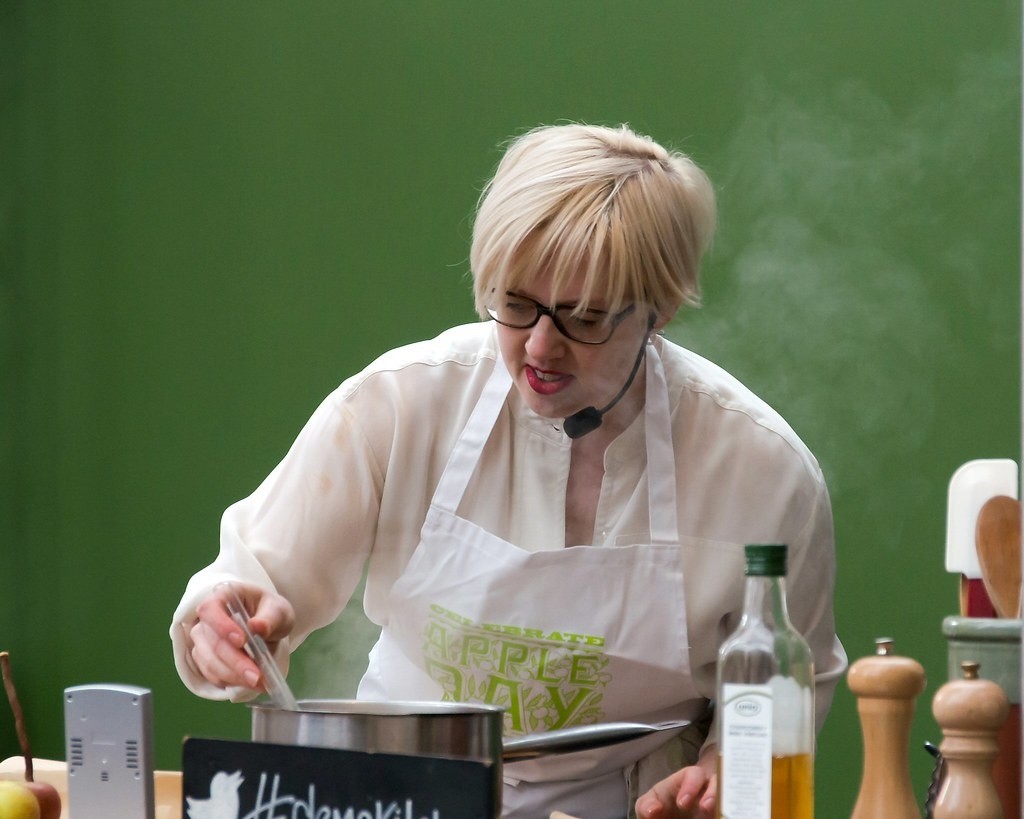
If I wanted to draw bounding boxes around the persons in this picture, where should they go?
[167,125,849,819]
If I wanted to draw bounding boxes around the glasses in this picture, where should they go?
[479,285,648,345]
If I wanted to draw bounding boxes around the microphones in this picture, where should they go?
[564,315,656,439]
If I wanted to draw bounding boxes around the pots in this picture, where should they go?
[246,698,692,819]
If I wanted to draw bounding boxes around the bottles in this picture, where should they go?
[712,541,817,819]
[846,639,924,819]
[931,661,1009,819]
